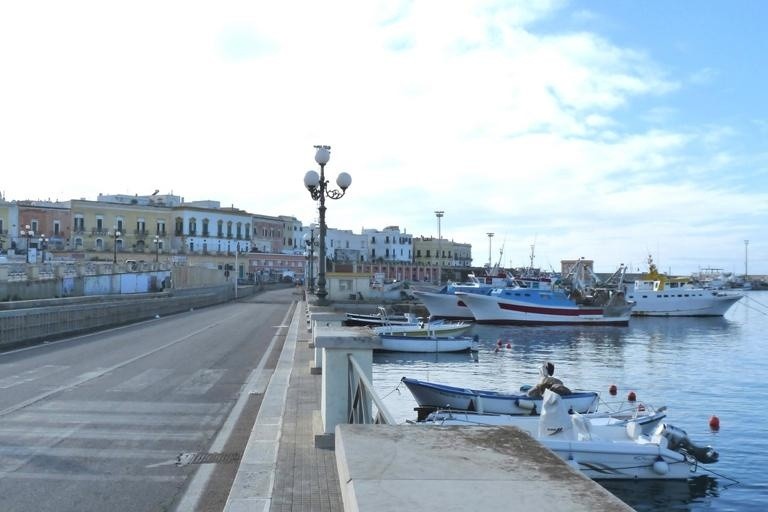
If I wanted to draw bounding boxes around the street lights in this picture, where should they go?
[38,233,49,263]
[153,235,162,263]
[304,145,351,305]
[20,224,34,264]
[107,225,120,265]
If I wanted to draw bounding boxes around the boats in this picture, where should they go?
[626,267,746,319]
[401,376,598,422]
[453,262,638,329]
[345,305,480,353]
[412,256,554,321]
[414,402,721,478]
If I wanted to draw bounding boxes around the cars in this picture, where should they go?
[293,277,302,286]
